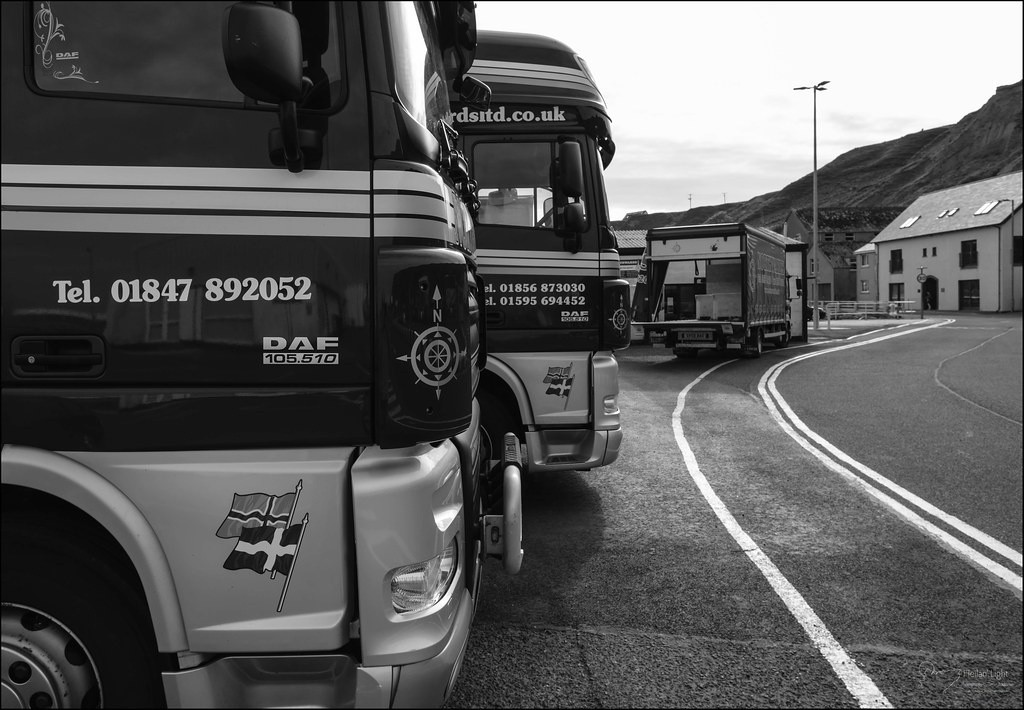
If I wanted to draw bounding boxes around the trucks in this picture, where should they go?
[632,225,792,359]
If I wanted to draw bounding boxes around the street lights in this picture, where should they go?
[973,199,1014,313]
[794,80,831,331]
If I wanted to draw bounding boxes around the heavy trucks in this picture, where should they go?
[450,29,632,513]
[0,0,526,710]
